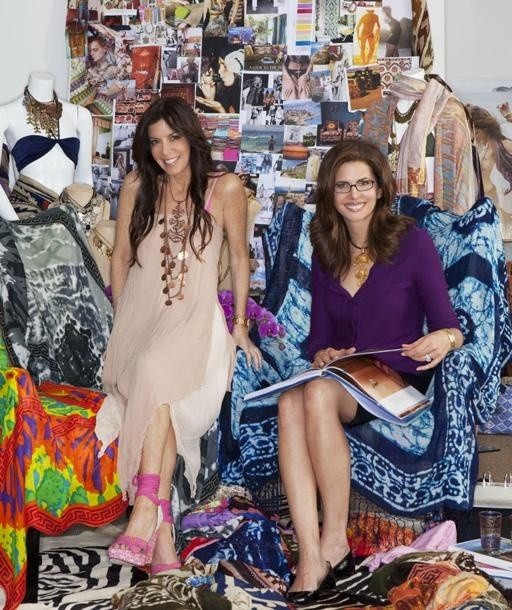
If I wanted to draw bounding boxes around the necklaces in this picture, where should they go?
[59,187,102,234]
[389,99,422,160]
[23,87,64,140]
[349,240,368,284]
[160,176,193,305]
[481,142,488,160]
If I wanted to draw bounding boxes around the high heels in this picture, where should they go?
[109,474,183,577]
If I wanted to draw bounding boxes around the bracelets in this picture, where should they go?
[232,317,247,327]
[443,327,456,351]
[504,111,511,117]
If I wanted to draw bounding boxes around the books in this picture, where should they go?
[242,347,431,425]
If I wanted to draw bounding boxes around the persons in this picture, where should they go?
[362,69,484,216]
[465,102,511,209]
[92,93,263,579]
[273,136,465,603]
[67,0,400,271]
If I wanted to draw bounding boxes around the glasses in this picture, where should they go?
[334,176,376,195]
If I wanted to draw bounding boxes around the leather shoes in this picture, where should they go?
[285,551,350,605]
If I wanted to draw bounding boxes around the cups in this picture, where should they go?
[479,510,503,553]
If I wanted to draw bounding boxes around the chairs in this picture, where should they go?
[217,196,512,520]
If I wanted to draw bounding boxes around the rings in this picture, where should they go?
[425,355,431,364]
